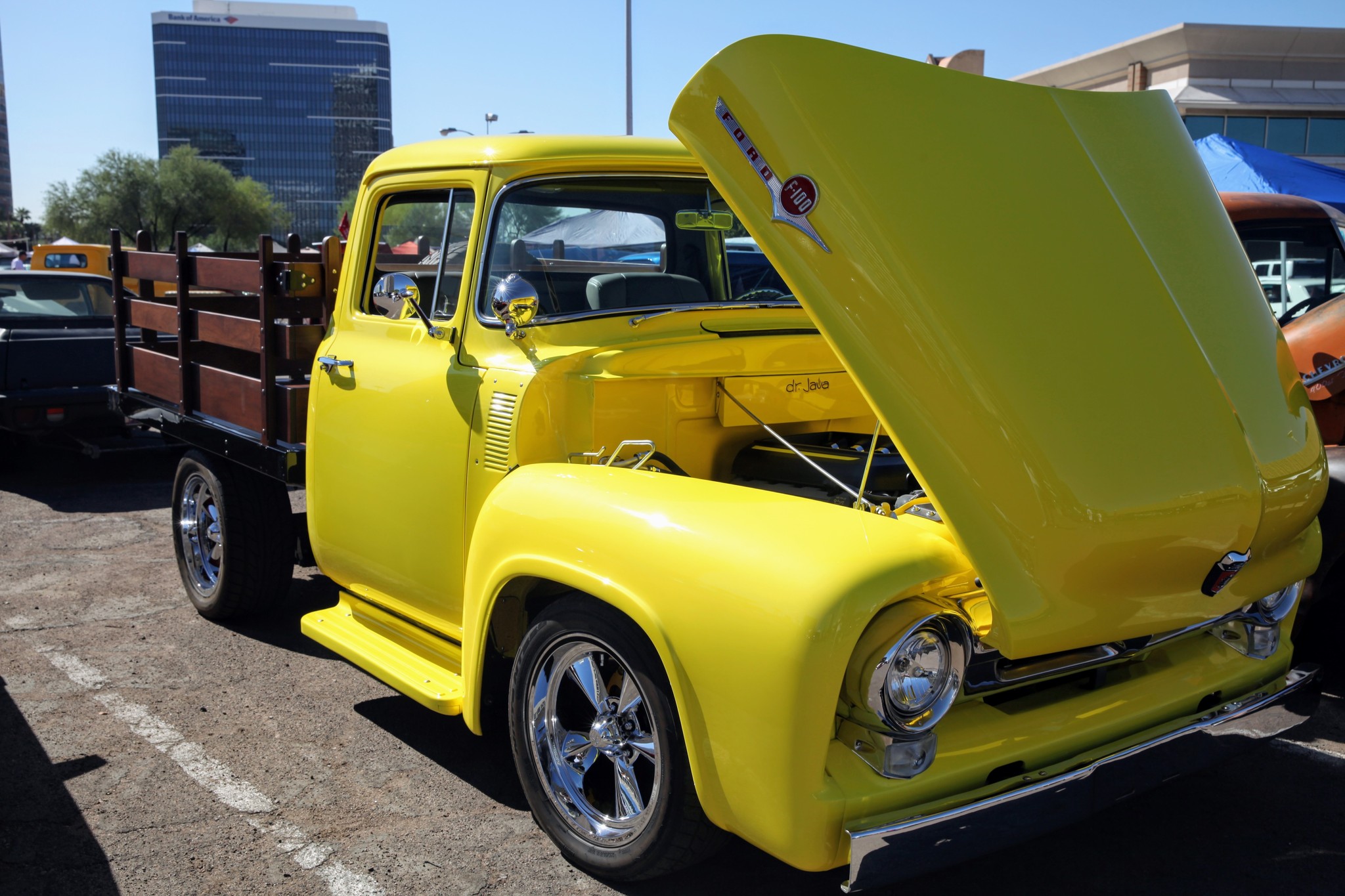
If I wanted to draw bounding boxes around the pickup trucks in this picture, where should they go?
[99,33,1332,895]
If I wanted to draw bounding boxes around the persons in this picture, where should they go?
[12,252,27,270]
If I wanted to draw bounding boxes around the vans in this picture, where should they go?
[30,243,198,316]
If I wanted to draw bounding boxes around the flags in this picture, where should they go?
[338,213,350,240]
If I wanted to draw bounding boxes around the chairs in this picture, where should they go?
[398,271,504,317]
[586,272,709,310]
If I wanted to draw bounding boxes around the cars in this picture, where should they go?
[0,268,180,435]
[1216,189,1345,715]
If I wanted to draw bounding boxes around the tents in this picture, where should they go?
[519,209,666,250]
[1193,134,1345,214]
